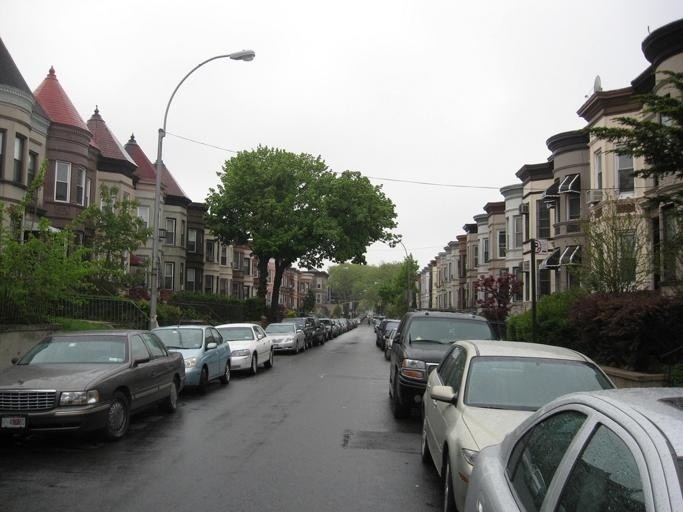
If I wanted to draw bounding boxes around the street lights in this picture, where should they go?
[147,50,255,331]
[393,233,413,312]
